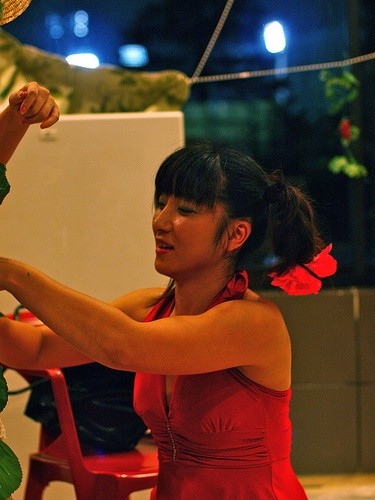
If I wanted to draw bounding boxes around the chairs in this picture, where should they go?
[4,312,160,500]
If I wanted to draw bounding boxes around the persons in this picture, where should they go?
[0,143,324,500]
